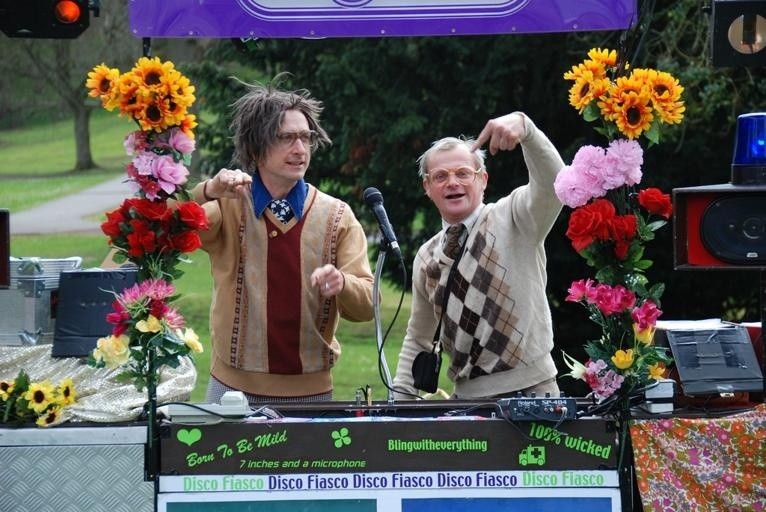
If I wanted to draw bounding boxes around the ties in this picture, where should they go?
[271,199,290,224]
[443,224,464,259]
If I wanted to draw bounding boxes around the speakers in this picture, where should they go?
[705,0,766,69]
[1,208,10,290]
[671,182,766,269]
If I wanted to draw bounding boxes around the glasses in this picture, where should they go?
[273,130,320,149]
[424,166,481,186]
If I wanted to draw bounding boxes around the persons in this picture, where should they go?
[388,110,568,402]
[165,70,384,406]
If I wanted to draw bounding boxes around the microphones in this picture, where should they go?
[362,186,404,263]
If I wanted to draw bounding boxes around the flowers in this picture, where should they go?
[554,48,686,472]
[84,56,213,451]
[0,369,77,428]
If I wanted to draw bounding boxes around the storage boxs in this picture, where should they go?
[1,257,83,346]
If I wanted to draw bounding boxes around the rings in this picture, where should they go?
[325,282,335,293]
